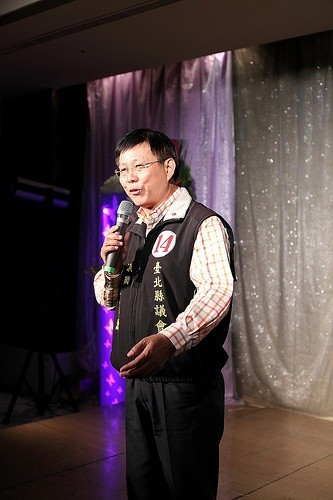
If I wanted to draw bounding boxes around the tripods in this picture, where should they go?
[3,345,80,426]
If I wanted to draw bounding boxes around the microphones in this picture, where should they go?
[105,200,133,273]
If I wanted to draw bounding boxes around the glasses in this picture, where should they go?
[115,159,161,177]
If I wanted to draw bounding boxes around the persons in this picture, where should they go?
[93,129,235,500]
